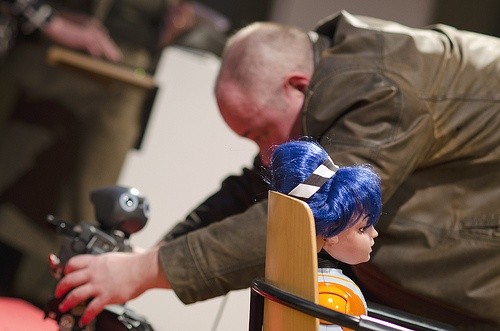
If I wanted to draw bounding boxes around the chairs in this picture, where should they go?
[248,190,447,331]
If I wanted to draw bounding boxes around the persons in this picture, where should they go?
[0,0,197,66]
[55,9,500,331]
[263,138,381,331]
